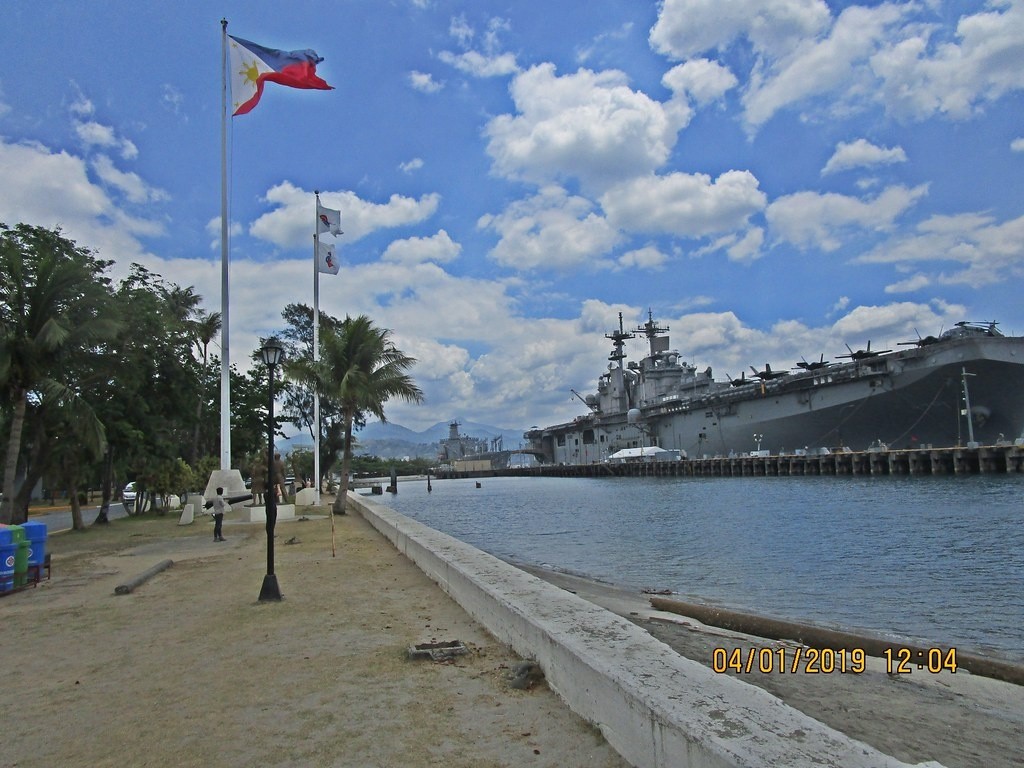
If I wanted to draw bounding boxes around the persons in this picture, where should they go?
[274,452,288,502]
[250,458,265,504]
[213,487,226,542]
[301,479,311,488]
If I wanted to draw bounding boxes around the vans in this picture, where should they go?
[123,482,138,506]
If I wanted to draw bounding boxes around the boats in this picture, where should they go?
[514,300,1024,464]
[434,418,514,470]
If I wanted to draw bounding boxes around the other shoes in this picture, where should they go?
[220,537,225,540]
[214,538,220,542]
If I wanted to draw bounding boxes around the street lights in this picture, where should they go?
[838,404,855,448]
[752,433,763,451]
[255,335,286,604]
[960,363,978,447]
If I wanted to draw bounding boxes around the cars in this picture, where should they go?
[245,477,252,488]
[285,476,296,485]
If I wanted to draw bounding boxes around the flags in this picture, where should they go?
[319,241,340,276]
[317,205,340,234]
[228,34,336,114]
